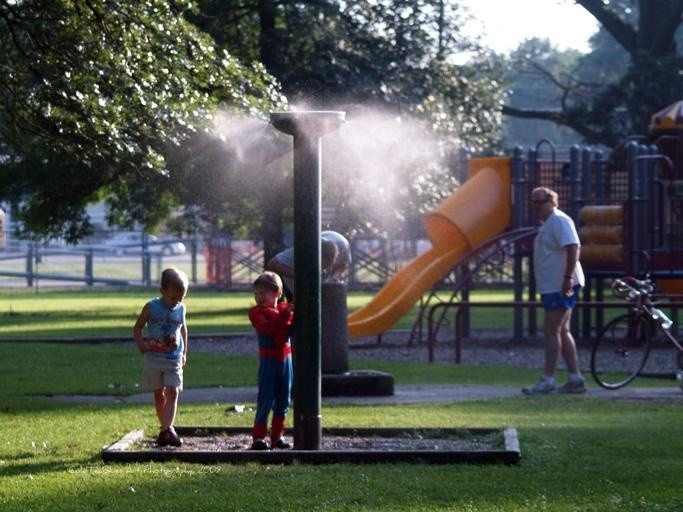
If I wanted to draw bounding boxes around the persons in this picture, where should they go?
[246,271,294,450]
[132,267,190,446]
[520,186,588,395]
[264,237,351,303]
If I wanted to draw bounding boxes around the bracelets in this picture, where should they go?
[564,273,575,280]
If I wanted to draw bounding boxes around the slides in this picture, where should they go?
[347,168,504,338]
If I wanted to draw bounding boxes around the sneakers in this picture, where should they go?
[555,378,588,394]
[521,377,556,395]
[271,436,291,449]
[251,438,268,450]
[156,425,183,448]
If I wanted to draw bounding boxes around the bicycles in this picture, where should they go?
[590,276,683,391]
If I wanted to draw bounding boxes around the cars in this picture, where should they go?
[102,230,187,256]
